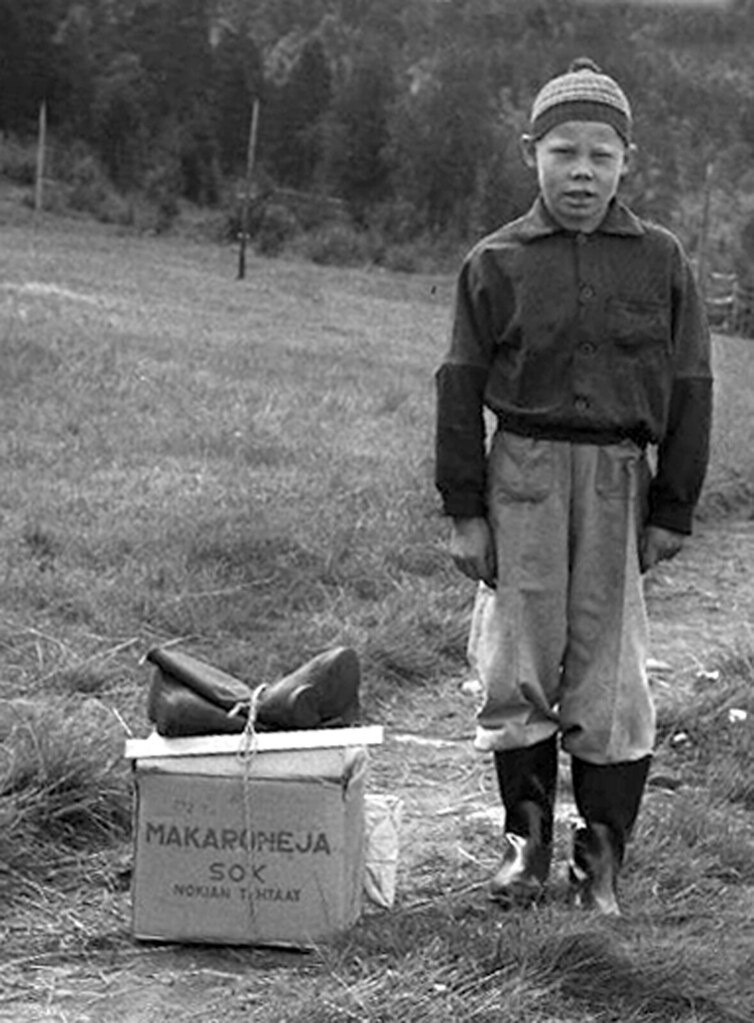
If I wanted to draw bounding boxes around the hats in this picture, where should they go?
[530,56,632,150]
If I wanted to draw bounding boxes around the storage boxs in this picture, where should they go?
[123,723,391,952]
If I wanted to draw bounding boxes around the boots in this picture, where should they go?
[484,731,558,905]
[146,645,359,729]
[148,671,348,738]
[567,753,653,922]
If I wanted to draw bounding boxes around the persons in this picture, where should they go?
[434,58,713,913]
